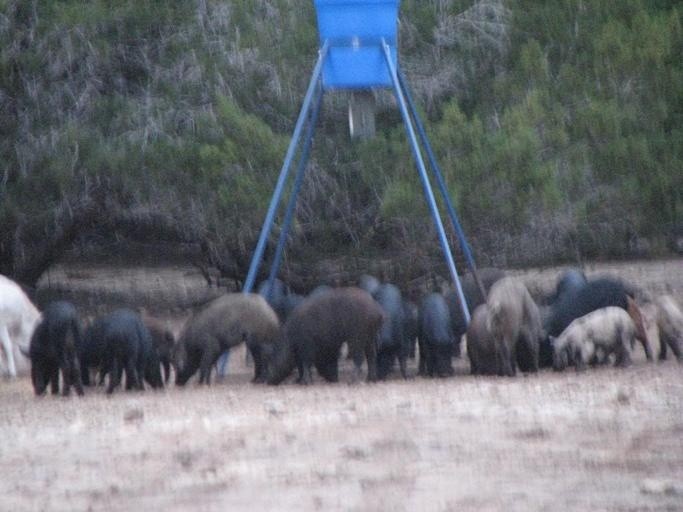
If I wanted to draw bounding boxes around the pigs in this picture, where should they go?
[0,265,683,397]
